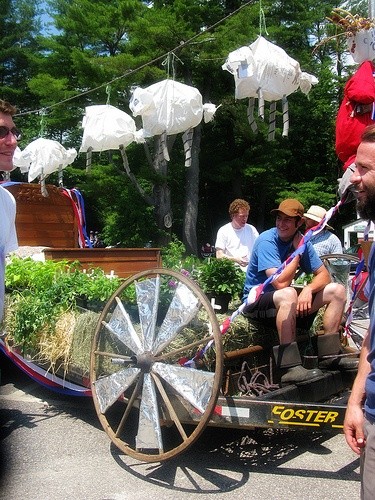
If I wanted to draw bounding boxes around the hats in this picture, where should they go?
[303,205,335,230]
[271,198,305,218]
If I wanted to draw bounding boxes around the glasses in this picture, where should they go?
[0,125,19,139]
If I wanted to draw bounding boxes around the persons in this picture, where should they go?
[241,199,360,388]
[215,199,259,272]
[303,204,344,265]
[343,126,375,500]
[0,97,22,323]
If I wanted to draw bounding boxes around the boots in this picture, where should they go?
[273,342,325,387]
[317,332,360,373]
